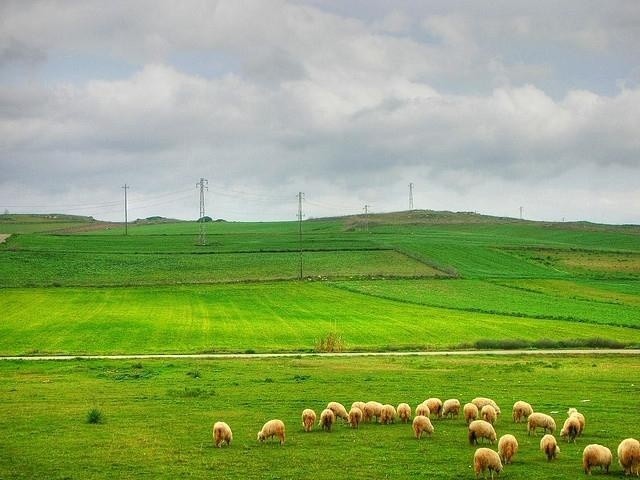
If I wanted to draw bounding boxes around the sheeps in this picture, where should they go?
[497,433,519,465]
[411,415,435,440]
[539,433,561,462]
[567,407,585,436]
[256,419,286,447]
[301,408,317,432]
[212,420,234,448]
[560,416,581,443]
[472,446,504,480]
[581,443,613,475]
[526,412,557,437]
[318,396,502,432]
[467,419,497,445]
[511,400,534,423]
[616,437,640,476]
